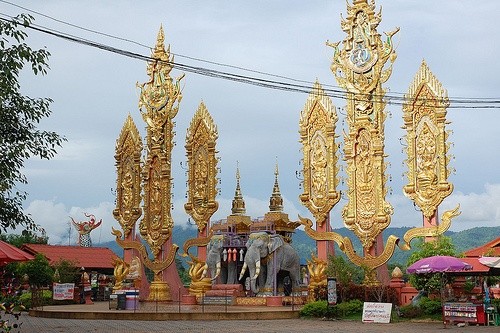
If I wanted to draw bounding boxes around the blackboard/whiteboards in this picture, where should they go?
[104,287,111,300]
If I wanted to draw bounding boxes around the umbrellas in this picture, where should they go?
[0,240,36,267]
[405,256,474,306]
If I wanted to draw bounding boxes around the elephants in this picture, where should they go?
[206,235,236,284]
[238,231,300,297]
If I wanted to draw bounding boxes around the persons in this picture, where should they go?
[91,276,97,287]
[283,276,293,297]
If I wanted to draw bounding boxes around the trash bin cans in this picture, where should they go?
[125,290,139,309]
[109,294,126,309]
